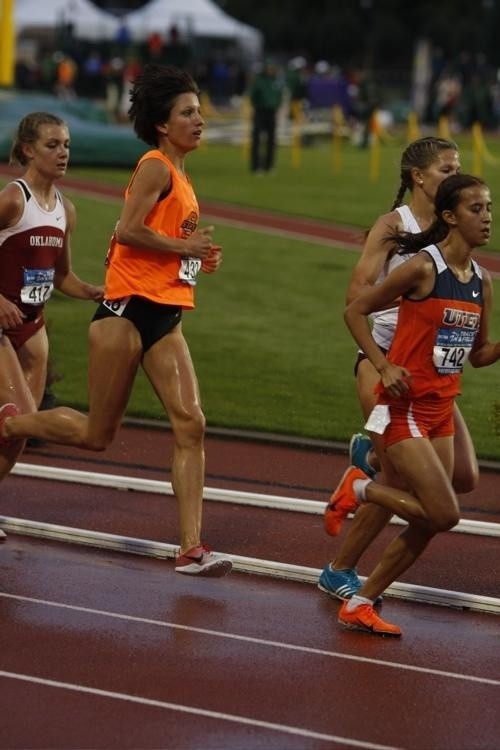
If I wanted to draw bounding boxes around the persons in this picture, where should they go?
[0,112,108,542]
[206,48,500,175]
[0,58,236,577]
[12,19,197,121]
[304,134,482,611]
[321,173,498,640]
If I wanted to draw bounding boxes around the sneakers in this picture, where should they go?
[0,403,19,449]
[324,465,367,537]
[176,546,232,578]
[317,562,384,605]
[338,600,401,638]
[349,433,376,476]
